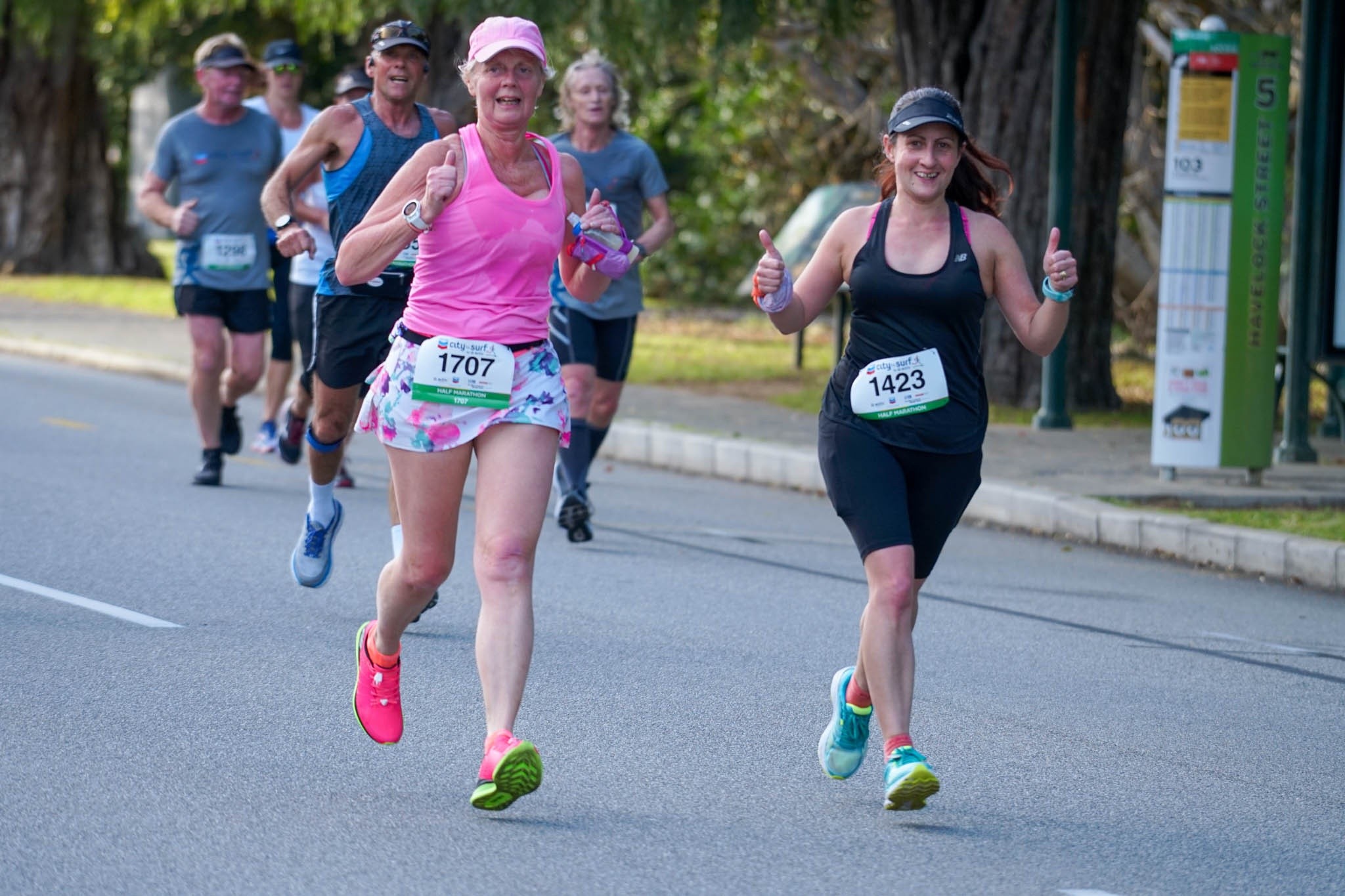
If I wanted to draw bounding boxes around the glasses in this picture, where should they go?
[273,62,301,75]
[370,25,429,44]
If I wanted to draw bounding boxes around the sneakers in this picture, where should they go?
[352,618,405,747]
[883,745,941,811]
[278,398,307,465]
[469,730,543,811]
[219,403,244,454]
[409,590,439,623]
[335,467,354,487]
[817,665,873,780]
[192,448,223,487]
[290,497,345,589]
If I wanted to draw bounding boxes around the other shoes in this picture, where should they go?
[553,462,595,543]
[251,428,277,454]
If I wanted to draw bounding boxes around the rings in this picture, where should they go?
[1059,271,1068,279]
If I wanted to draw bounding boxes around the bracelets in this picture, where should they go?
[631,240,647,258]
[1042,276,1073,302]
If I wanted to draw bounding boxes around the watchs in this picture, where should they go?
[402,198,433,234]
[273,214,297,231]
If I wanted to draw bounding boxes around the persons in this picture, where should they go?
[279,65,372,488]
[240,42,327,454]
[136,33,283,486]
[753,88,1080,809]
[544,57,676,543]
[260,20,458,623]
[334,18,620,810]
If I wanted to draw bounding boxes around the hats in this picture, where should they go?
[370,20,431,57]
[887,95,964,136]
[201,44,258,71]
[262,40,305,69]
[467,15,547,70]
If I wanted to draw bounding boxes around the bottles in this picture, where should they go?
[566,212,639,265]
